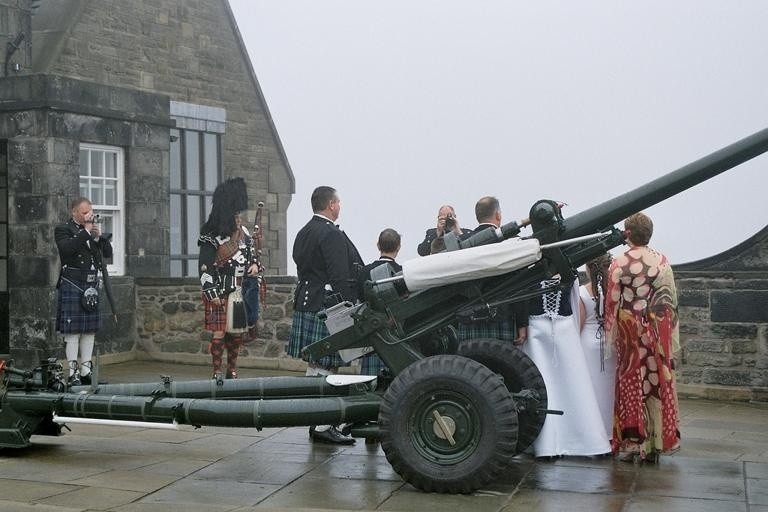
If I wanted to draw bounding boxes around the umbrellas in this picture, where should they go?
[92,215,119,326]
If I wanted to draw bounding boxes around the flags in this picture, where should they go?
[248,206,263,254]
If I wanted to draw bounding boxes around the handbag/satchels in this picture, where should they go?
[80,287,100,313]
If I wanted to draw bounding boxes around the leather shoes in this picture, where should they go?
[80,371,109,385]
[67,370,81,386]
[308,424,357,445]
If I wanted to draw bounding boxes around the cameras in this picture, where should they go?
[91,213,100,223]
[444,212,454,229]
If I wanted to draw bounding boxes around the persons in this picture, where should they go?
[285,185,365,446]
[195,206,259,381]
[356,228,408,445]
[575,253,618,441]
[602,212,681,465]
[457,195,531,347]
[516,198,611,463]
[54,196,113,388]
[416,205,474,258]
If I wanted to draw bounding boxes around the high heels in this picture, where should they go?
[620,450,640,464]
[642,451,660,465]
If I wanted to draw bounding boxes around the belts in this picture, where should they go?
[215,266,247,278]
[65,268,98,284]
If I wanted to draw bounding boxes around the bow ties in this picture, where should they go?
[76,224,84,229]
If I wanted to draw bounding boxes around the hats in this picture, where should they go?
[210,176,248,216]
